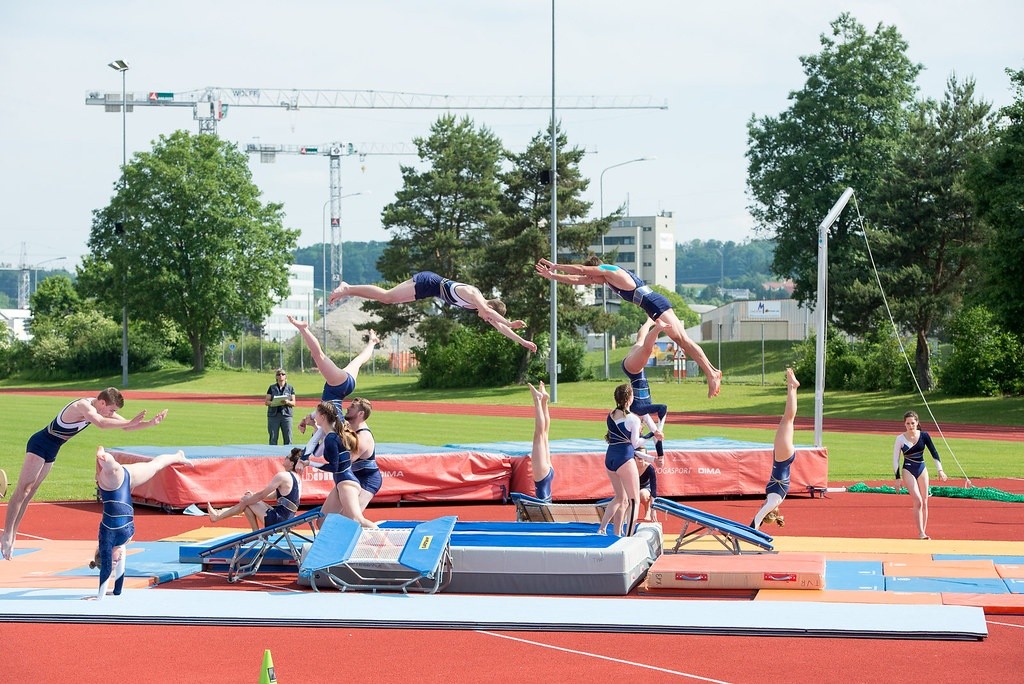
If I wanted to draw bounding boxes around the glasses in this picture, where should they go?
[277,373,285,376]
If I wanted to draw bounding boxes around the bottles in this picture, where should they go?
[259,650,276,684]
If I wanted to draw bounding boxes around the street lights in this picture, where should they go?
[600,158,647,380]
[322,192,364,352]
[34,257,68,294]
[110,58,130,387]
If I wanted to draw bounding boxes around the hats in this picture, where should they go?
[276,368,285,373]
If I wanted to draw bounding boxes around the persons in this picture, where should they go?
[81,445,194,603]
[527,380,554,505]
[535,256,722,400]
[265,368,296,446]
[329,271,537,353]
[749,368,800,530]
[596,316,673,537]
[2,388,169,561]
[206,447,304,533]
[893,411,948,540]
[287,316,395,546]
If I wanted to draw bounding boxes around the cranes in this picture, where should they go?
[242,140,600,308]
[86,84,669,140]
